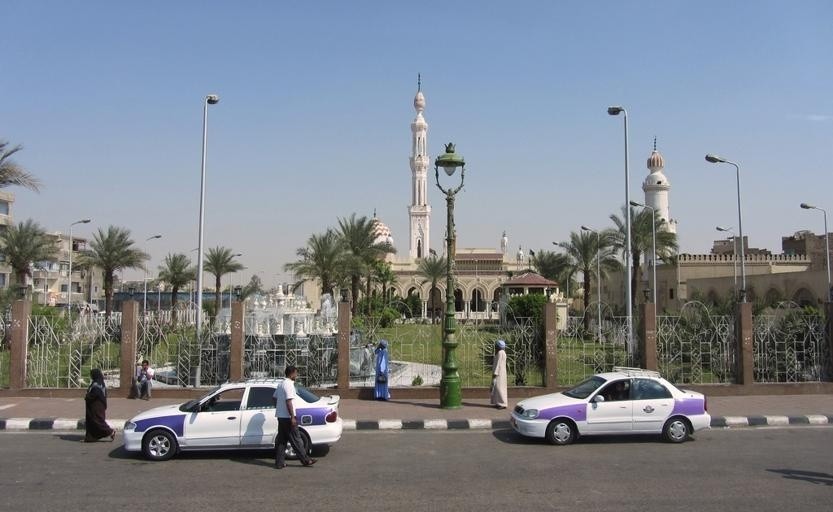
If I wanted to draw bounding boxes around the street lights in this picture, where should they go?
[796,200,832,303]
[188,247,200,312]
[606,103,636,354]
[191,91,221,386]
[703,151,748,302]
[433,139,469,411]
[228,251,243,317]
[42,239,62,307]
[712,224,736,297]
[143,232,163,327]
[549,195,739,358]
[65,215,92,322]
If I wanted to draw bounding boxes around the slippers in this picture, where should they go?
[111,431,115,440]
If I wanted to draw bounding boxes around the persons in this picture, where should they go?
[272,367,318,470]
[372,340,392,400]
[490,340,508,410]
[78,368,116,443]
[132,360,154,401]
[606,381,628,400]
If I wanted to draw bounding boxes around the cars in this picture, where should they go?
[505,364,716,447]
[120,374,345,464]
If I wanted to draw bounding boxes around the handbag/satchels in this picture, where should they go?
[377,375,386,383]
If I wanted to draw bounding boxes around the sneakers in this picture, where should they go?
[134,396,152,400]
[283,464,287,467]
[303,459,317,466]
